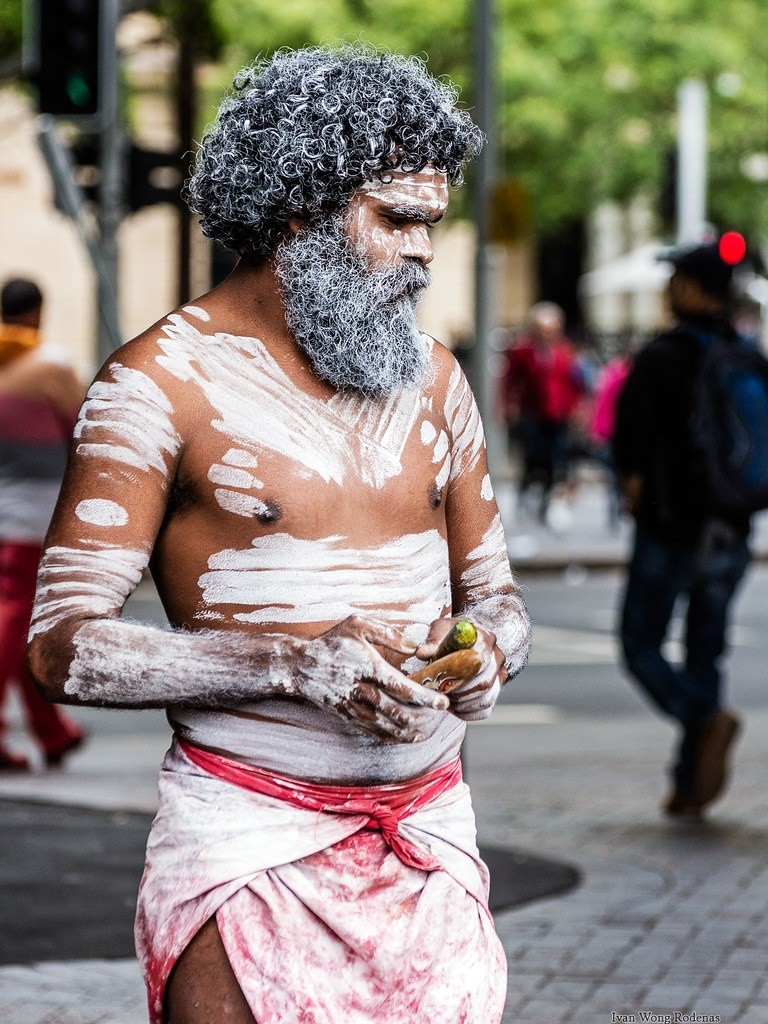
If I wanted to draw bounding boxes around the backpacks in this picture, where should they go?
[652,327,768,518]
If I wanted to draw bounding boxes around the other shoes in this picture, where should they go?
[44,725,87,768]
[0,750,29,772]
[688,712,742,810]
[661,788,700,818]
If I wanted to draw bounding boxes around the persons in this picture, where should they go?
[608,240,768,819]
[29,44,529,1024]
[500,303,587,523]
[1,280,87,773]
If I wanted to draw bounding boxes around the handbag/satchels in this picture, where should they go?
[565,423,592,459]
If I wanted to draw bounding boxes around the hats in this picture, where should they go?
[656,242,734,305]
